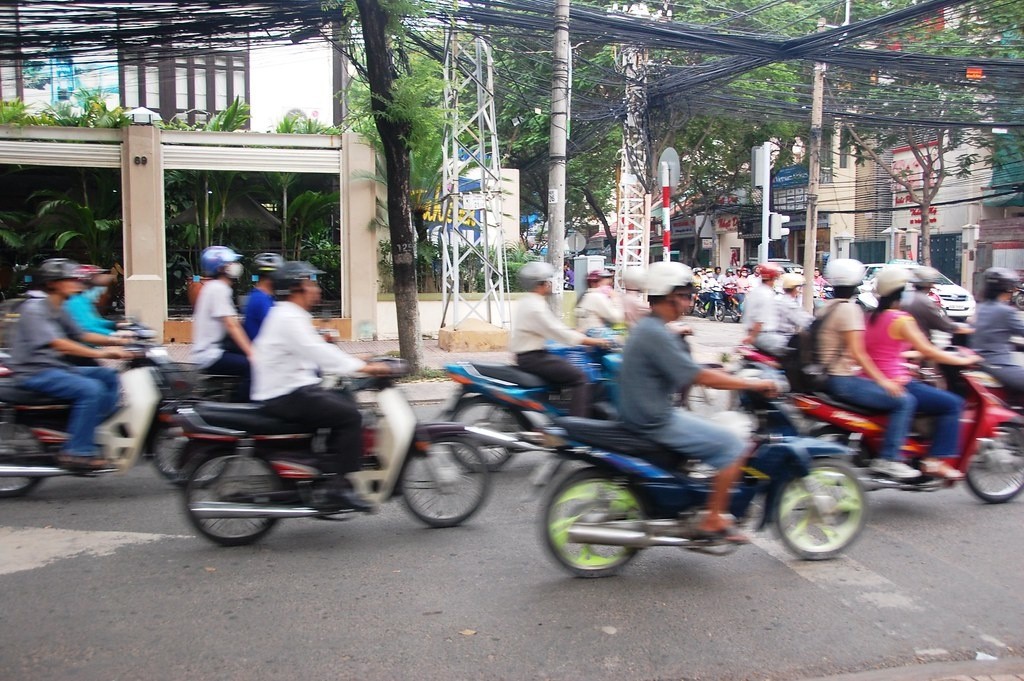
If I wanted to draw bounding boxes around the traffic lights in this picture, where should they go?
[771,214,790,239]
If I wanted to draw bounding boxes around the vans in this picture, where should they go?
[861,265,976,322]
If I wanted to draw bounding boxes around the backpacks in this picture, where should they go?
[789,299,850,381]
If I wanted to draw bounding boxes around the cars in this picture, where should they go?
[743,262,802,275]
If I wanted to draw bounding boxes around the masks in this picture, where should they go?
[223,261,243,279]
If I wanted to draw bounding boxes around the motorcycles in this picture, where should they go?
[0,311,240,500]
[152,326,492,547]
[1012,284,1024,311]
[438,320,1023,579]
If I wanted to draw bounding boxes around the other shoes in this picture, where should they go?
[870,457,923,479]
[61,455,110,471]
[327,491,378,513]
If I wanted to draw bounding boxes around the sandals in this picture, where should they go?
[921,459,967,482]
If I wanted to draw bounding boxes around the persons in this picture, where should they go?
[617,261,780,544]
[4,260,136,468]
[191,245,392,511]
[509,257,1024,481]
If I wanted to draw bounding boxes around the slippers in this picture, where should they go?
[691,522,747,542]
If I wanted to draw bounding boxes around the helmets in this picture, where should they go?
[783,274,806,292]
[250,254,285,275]
[914,266,937,284]
[877,265,907,297]
[78,263,108,281]
[519,261,553,290]
[693,266,758,277]
[985,266,1021,285]
[200,246,243,274]
[646,261,695,296]
[623,265,646,290]
[586,269,613,284]
[824,258,866,287]
[758,263,785,279]
[39,257,88,285]
[281,259,327,282]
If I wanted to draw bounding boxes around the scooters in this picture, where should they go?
[692,285,836,321]
